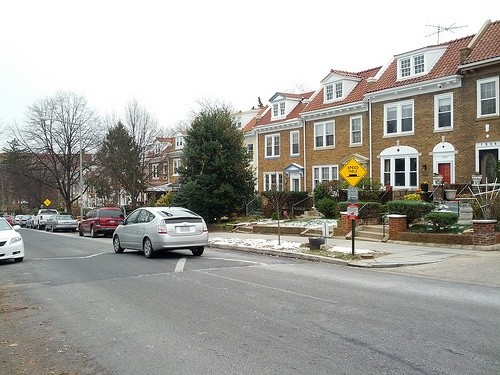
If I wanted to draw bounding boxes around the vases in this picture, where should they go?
[444,190,457,201]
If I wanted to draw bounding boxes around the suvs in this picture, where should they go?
[78,207,126,238]
[35,208,60,230]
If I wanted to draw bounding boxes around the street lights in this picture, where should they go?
[38,116,84,218]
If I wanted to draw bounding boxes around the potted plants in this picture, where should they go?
[421,181,429,191]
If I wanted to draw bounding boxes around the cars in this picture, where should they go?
[2,213,38,228]
[47,215,80,233]
[0,218,25,263]
[112,206,209,258]
